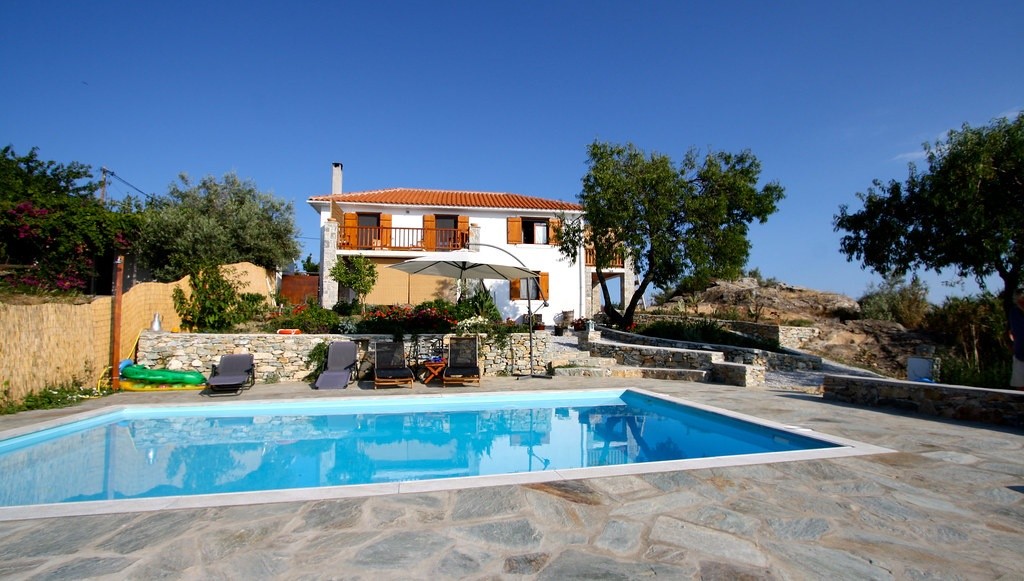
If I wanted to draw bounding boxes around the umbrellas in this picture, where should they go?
[384,246,541,301]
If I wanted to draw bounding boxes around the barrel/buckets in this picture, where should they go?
[562,310,574,326]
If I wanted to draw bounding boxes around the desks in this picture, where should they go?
[424,361,445,384]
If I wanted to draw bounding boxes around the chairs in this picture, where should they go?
[374,342,415,389]
[442,337,481,388]
[314,341,358,390]
[208,354,256,397]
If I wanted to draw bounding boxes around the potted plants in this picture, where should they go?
[535,322,546,330]
[572,317,587,332]
[554,324,568,336]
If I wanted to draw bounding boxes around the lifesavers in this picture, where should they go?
[276,328,302,334]
